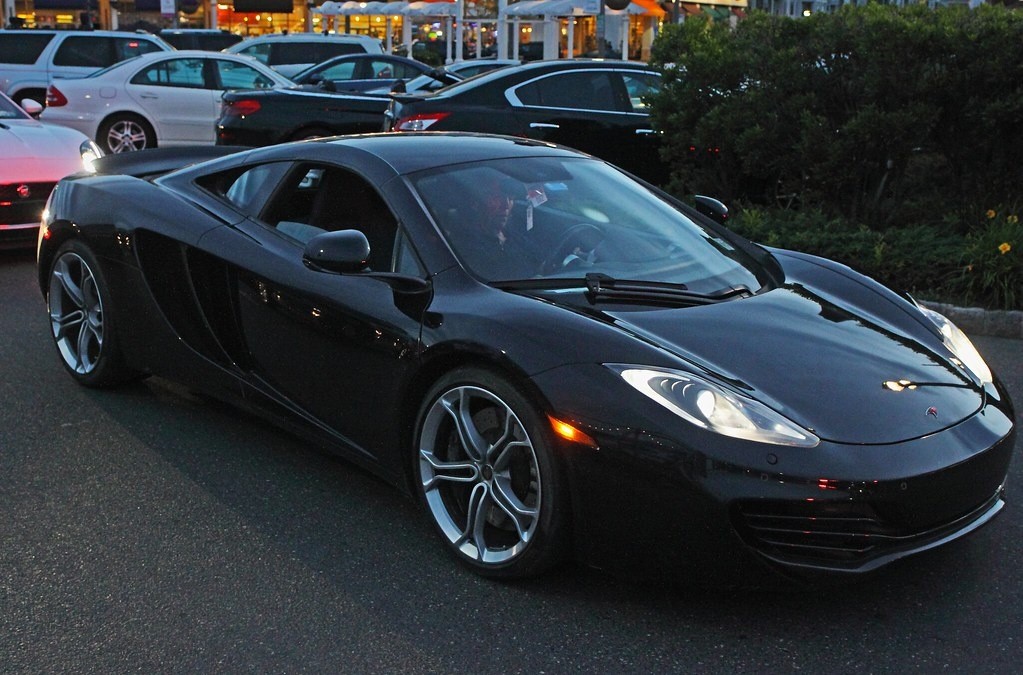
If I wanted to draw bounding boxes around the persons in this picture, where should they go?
[451,175,597,277]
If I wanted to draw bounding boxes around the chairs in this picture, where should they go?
[309,166,374,264]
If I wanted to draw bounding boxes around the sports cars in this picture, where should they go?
[34,129,1017,589]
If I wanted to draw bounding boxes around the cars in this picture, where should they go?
[384,60,735,185]
[212,77,456,157]
[0,89,104,247]
[0,26,526,163]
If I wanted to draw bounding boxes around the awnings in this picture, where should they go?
[662,2,746,19]
